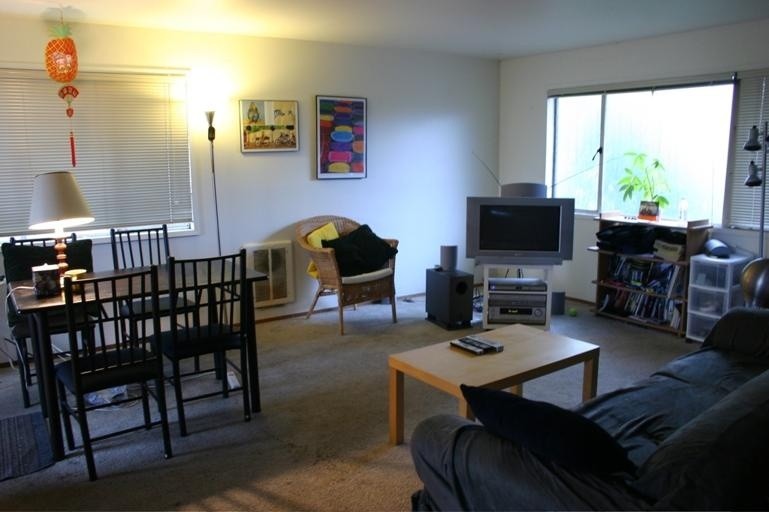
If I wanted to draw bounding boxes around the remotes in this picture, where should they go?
[470,335,504,353]
[460,337,492,353]
[451,339,482,356]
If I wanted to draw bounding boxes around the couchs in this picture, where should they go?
[408,308,767,512]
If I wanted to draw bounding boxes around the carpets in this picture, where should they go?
[0,411,57,483]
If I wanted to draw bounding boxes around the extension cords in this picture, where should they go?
[227,371,242,391]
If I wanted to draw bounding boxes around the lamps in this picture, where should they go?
[743,124,763,152]
[27,171,95,291]
[744,160,763,187]
[171,71,238,256]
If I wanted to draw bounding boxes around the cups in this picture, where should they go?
[65,268,87,294]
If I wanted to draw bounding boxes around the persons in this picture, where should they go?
[248,103,259,122]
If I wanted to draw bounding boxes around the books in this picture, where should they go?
[598,255,683,331]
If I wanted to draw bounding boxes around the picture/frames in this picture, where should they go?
[239,99,299,153]
[315,95,367,180]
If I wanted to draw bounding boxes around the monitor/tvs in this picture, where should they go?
[465,195,576,268]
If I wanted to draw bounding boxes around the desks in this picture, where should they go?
[388,323,600,446]
[8,256,268,460]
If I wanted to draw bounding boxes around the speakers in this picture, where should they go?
[440,244,458,271]
[552,291,566,315]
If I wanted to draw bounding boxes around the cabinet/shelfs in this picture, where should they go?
[482,264,552,331]
[686,248,759,344]
[587,211,714,338]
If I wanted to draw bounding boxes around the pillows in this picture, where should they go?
[321,224,398,277]
[460,383,638,478]
[1,239,99,328]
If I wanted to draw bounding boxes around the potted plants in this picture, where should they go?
[617,148,670,221]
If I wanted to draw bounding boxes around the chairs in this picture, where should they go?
[294,215,399,336]
[149,249,251,436]
[109,224,199,373]
[55,264,172,482]
[7,233,95,408]
